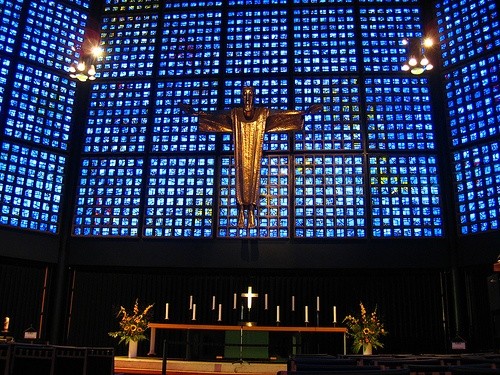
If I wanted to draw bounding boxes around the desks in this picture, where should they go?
[147,323,347,356]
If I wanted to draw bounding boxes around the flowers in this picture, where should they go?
[342,302,388,353]
[109,301,153,346]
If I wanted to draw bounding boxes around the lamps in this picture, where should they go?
[69,45,96,81]
[402,36,434,74]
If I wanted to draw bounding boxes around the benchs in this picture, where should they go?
[277,353,500,375]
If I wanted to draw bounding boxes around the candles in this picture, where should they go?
[277,306,279,321]
[190,296,192,309]
[166,303,168,319]
[265,294,268,309]
[219,304,221,320]
[213,296,215,309]
[4,317,9,330]
[334,306,336,322]
[305,306,308,322]
[193,304,195,320]
[292,296,294,311]
[317,297,319,311]
[240,306,243,319]
[234,294,236,309]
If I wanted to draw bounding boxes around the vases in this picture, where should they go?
[363,341,372,356]
[128,339,138,358]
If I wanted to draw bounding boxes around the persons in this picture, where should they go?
[180,88,324,227]
